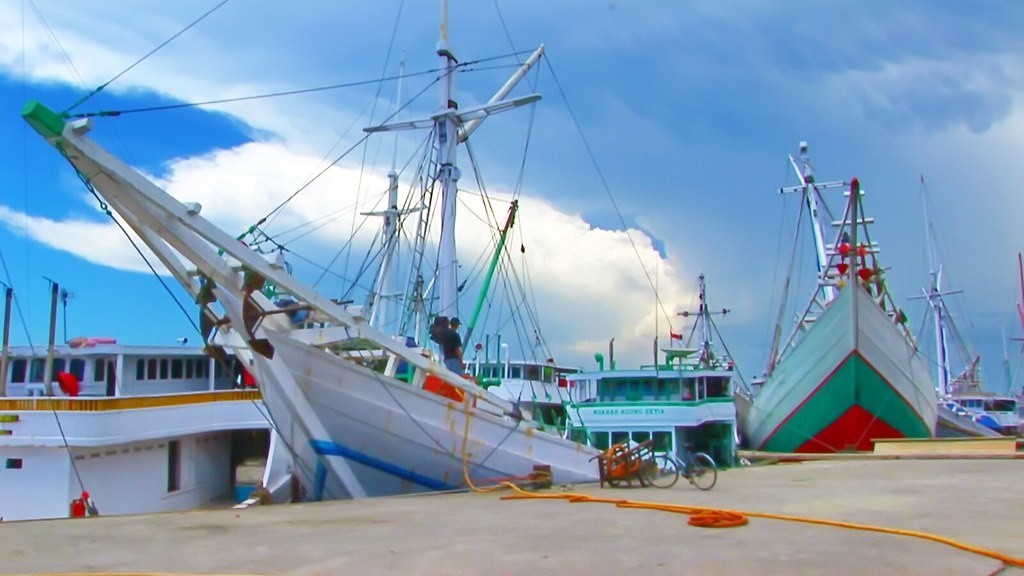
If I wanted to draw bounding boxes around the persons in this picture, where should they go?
[442,318,467,375]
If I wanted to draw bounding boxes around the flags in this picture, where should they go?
[458,280,467,292]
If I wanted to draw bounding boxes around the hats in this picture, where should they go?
[449,317,462,324]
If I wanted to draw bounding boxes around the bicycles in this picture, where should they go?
[636,435,718,491]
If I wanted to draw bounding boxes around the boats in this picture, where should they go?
[0,1,1024,524]
